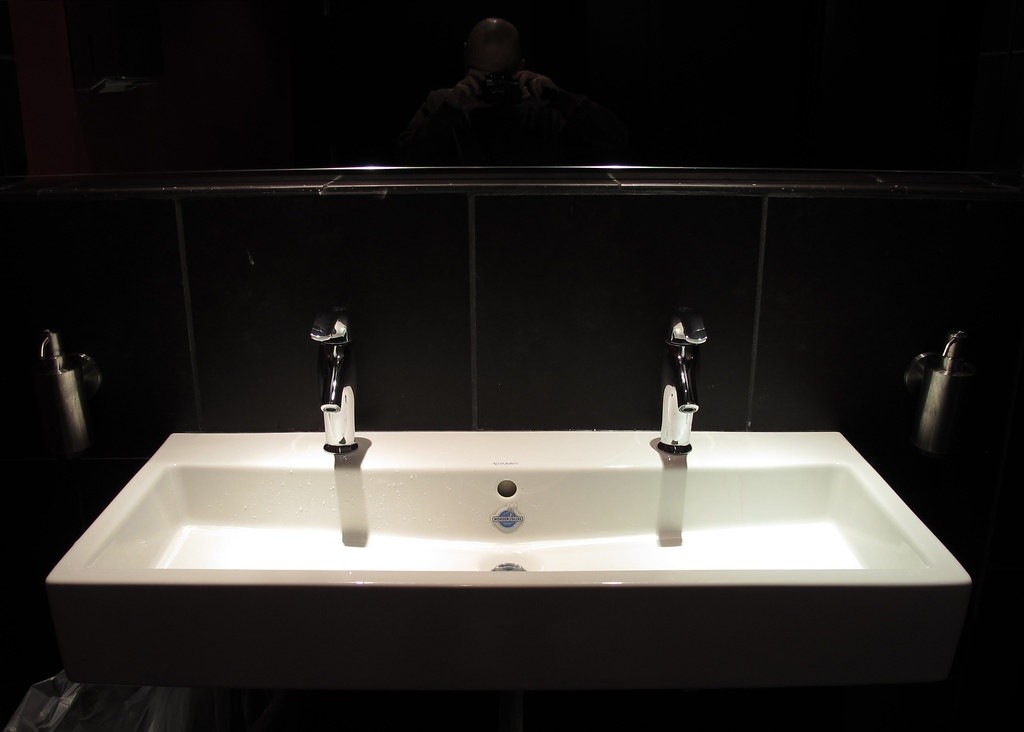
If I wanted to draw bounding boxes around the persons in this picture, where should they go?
[400,19,628,166]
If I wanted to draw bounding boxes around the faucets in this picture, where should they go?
[310,302,359,452]
[656,308,708,455]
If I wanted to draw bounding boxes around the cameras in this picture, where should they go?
[477,70,532,105]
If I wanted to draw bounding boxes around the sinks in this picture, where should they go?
[44,430,973,683]
